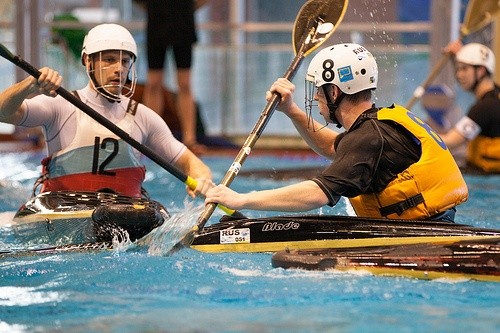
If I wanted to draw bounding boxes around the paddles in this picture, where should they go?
[165,0,348,257]
[0,43,248,221]
[405,0,500,111]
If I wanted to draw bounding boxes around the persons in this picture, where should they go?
[204,42,469,222]
[435,40,500,175]
[0,23,217,202]
[135,0,210,157]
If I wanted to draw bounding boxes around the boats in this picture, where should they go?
[0,133,500,284]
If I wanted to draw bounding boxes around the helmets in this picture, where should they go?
[456,43,495,74]
[81,23,137,66]
[305,42,378,94]
